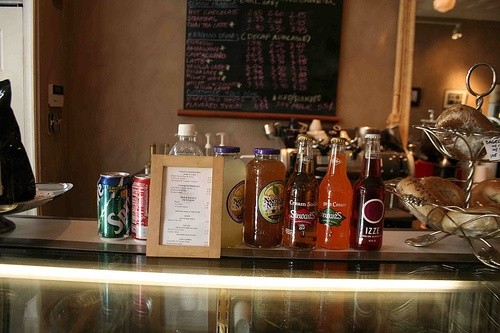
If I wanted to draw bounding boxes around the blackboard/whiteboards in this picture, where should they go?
[177,0,349,122]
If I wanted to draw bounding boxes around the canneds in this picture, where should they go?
[97,171,131,240]
[131,174,150,241]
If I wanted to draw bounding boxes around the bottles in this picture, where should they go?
[315,139,353,252]
[281,138,319,249]
[169,124,204,156]
[244,148,286,248]
[213,145,245,247]
[350,133,385,250]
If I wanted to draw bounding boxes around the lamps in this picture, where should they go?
[448,27,464,41]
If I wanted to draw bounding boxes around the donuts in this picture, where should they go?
[397,103,500,235]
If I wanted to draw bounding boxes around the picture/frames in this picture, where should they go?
[443,90,468,108]
